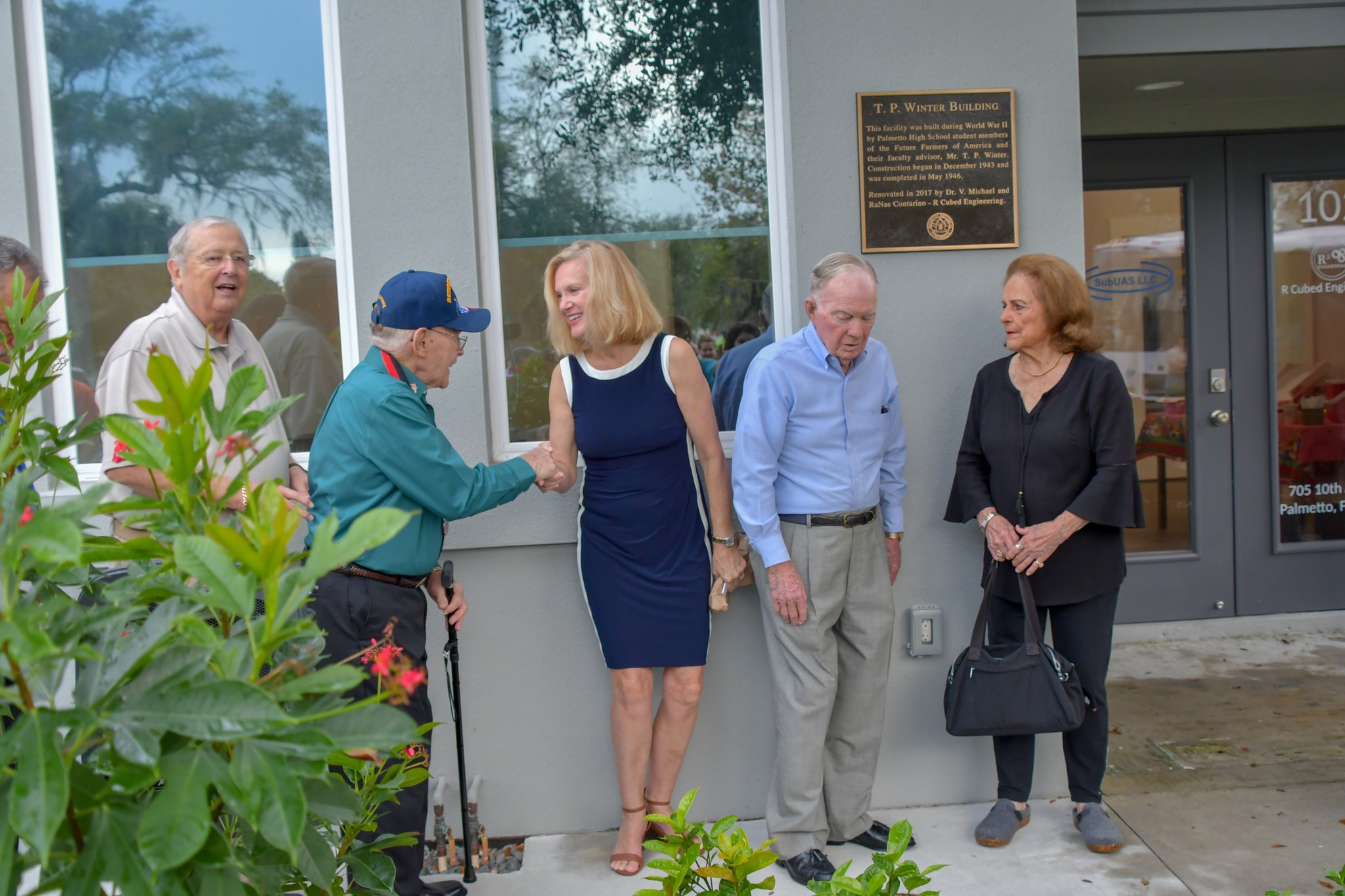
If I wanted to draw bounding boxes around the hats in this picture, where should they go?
[370,269,492,333]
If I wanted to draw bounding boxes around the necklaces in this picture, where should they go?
[1018,348,1064,377]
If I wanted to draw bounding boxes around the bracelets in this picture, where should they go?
[242,482,248,514]
[289,463,309,475]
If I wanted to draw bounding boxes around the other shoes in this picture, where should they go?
[1072,801,1126,853]
[974,797,1033,846]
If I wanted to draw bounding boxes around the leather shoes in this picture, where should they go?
[421,879,468,896]
[827,820,917,851]
[774,848,837,888]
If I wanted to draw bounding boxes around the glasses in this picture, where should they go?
[184,251,259,269]
[409,324,468,351]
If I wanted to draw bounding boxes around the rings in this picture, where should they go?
[1035,560,1044,568]
[1015,542,1024,551]
[996,550,1003,558]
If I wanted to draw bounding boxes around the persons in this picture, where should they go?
[94,215,315,558]
[661,315,721,393]
[300,270,556,896]
[256,256,344,453]
[944,255,1147,853]
[698,332,720,361]
[723,322,761,353]
[732,253,915,884]
[504,345,560,380]
[535,241,747,876]
[236,293,289,342]
[710,281,775,432]
[0,235,49,510]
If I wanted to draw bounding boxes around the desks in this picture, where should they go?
[1132,397,1345,531]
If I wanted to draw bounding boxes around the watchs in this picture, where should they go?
[981,511,999,534]
[712,536,737,547]
[885,532,904,541]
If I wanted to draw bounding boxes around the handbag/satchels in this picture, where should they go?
[940,552,1086,737]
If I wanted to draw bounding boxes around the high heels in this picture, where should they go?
[642,786,705,856]
[609,802,650,876]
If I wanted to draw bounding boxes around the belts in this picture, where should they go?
[776,508,883,528]
[331,565,429,591]
